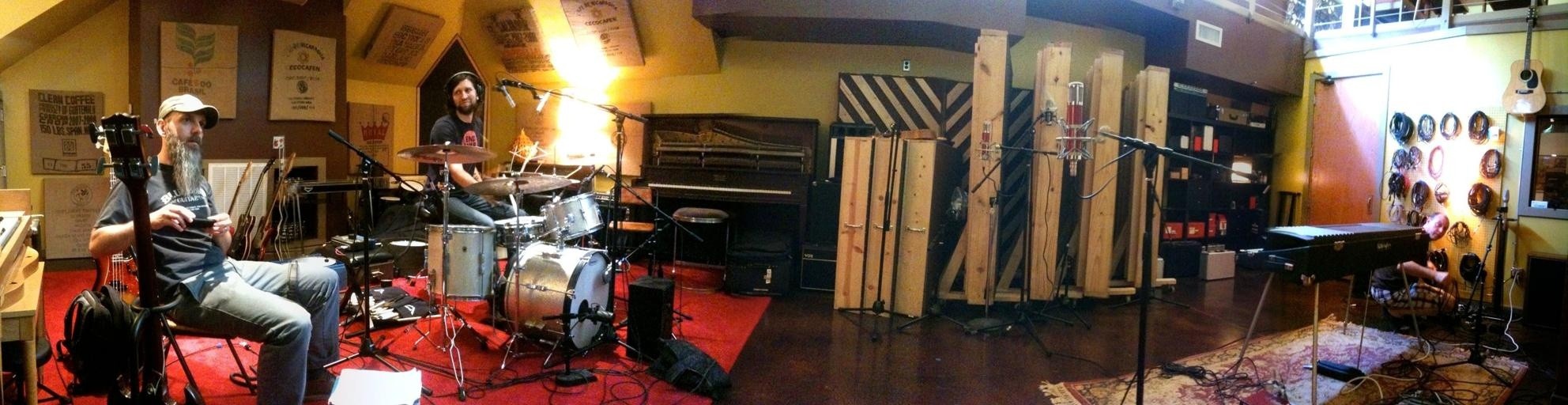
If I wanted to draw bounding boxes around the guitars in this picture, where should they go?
[228,147,280,262]
[1501,0,1547,117]
[89,244,138,309]
[224,160,253,254]
[87,113,204,405]
[253,152,298,260]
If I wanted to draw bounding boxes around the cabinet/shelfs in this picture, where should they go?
[1156,108,1275,277]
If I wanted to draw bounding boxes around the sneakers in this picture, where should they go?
[304,369,336,401]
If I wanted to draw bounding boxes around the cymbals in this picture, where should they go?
[464,174,579,197]
[397,143,497,164]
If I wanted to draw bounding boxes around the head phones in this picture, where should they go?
[442,71,484,108]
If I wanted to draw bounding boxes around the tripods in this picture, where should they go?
[501,195,550,369]
[838,123,918,343]
[976,144,1054,356]
[1429,208,1512,390]
[501,79,657,364]
[379,153,490,401]
[600,163,704,326]
[323,128,433,396]
[1023,243,1092,331]
[897,303,972,337]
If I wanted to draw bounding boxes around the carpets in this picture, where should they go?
[2,257,773,404]
[1040,310,1535,404]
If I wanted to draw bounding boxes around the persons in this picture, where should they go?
[1370,210,1457,323]
[89,92,339,405]
[425,73,530,227]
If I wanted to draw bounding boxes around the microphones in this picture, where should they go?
[1044,100,1057,126]
[585,305,615,325]
[581,164,605,187]
[1502,190,1509,230]
[495,77,515,108]
[1065,82,1085,177]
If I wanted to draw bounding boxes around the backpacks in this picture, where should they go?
[55,285,134,393]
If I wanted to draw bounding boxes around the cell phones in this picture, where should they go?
[178,218,217,229]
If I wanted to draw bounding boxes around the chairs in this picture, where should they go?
[129,244,255,404]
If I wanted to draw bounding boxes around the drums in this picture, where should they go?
[493,213,547,249]
[425,223,497,302]
[540,193,605,244]
[388,240,428,273]
[398,180,426,204]
[381,195,401,213]
[503,238,612,353]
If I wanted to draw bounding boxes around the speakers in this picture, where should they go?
[806,181,841,249]
[827,123,875,183]
[626,275,674,362]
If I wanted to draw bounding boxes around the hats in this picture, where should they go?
[158,93,219,129]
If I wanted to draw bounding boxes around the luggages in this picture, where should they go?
[724,230,800,297]
